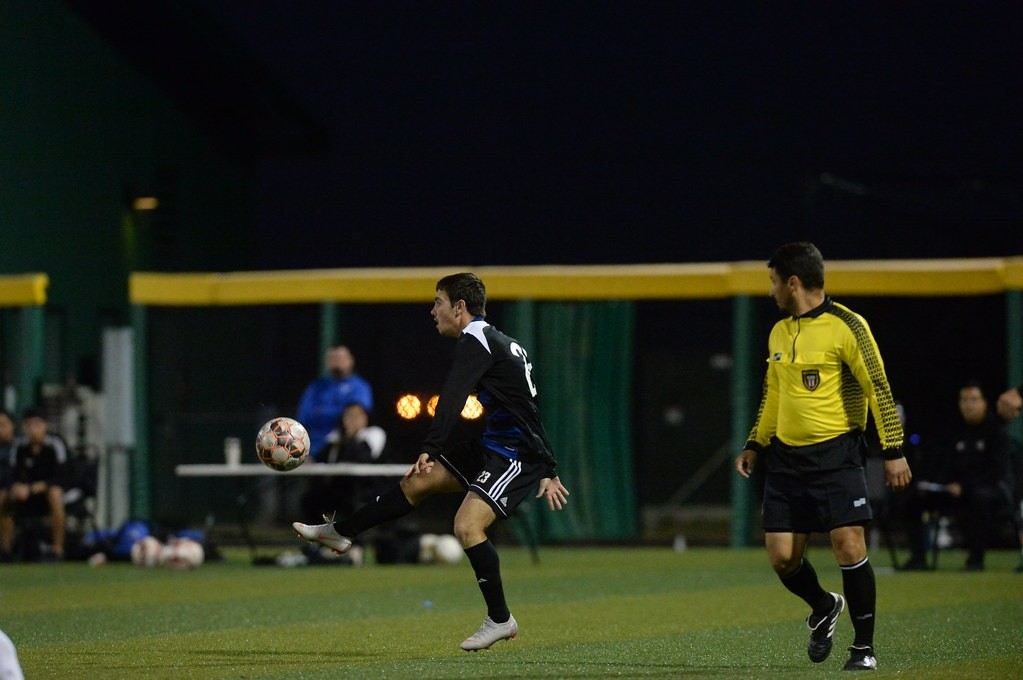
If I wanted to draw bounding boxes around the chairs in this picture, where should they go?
[863,432,945,568]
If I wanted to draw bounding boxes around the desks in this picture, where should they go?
[176,458,542,568]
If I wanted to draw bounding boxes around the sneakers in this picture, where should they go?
[292,511,351,556]
[460,613,518,652]
[843,646,877,670]
[805,592,844,662]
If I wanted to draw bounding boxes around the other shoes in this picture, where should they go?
[966,559,981,572]
[903,558,928,570]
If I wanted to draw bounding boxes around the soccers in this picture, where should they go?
[130,536,205,571]
[418,532,466,566]
[255,416,312,472]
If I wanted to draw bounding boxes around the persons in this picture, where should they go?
[0,340,388,569]
[899,380,1022,574]
[734,243,912,670]
[293,273,569,650]
